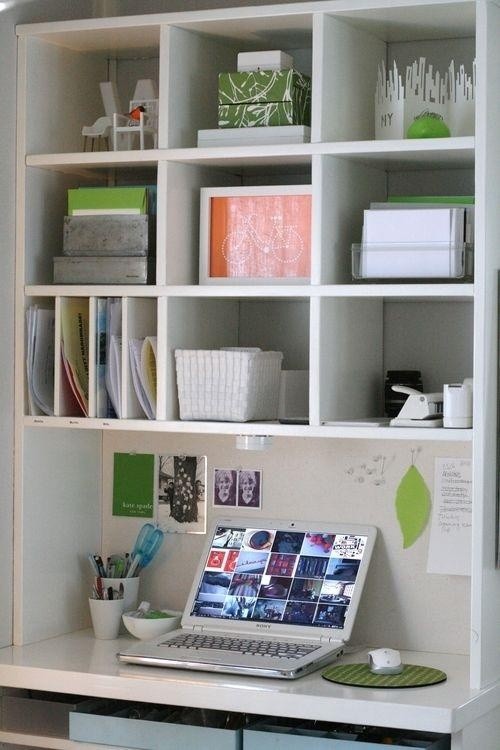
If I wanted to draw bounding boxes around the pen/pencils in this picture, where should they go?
[92,577,124,600]
[89,550,144,577]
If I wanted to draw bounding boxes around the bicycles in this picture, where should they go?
[220,206,305,267]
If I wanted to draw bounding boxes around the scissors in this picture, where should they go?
[131,523,163,577]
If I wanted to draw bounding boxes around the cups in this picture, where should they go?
[88,597,123,639]
[95,576,138,633]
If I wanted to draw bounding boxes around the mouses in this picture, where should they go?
[367,648,403,675]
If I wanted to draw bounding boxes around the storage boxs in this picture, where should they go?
[60,215,154,256]
[218,69,314,126]
[350,231,472,281]
[278,369,309,422]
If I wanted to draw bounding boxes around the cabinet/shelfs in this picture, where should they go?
[14,0,499,443]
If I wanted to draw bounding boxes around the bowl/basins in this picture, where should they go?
[122,610,183,639]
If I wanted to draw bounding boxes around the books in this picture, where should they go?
[25,297,157,419]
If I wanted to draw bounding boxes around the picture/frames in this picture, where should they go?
[196,181,311,285]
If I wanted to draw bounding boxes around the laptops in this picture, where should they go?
[116,516,379,680]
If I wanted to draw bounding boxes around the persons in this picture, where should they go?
[162,481,174,516]
[193,479,203,522]
[238,470,259,506]
[213,469,236,505]
